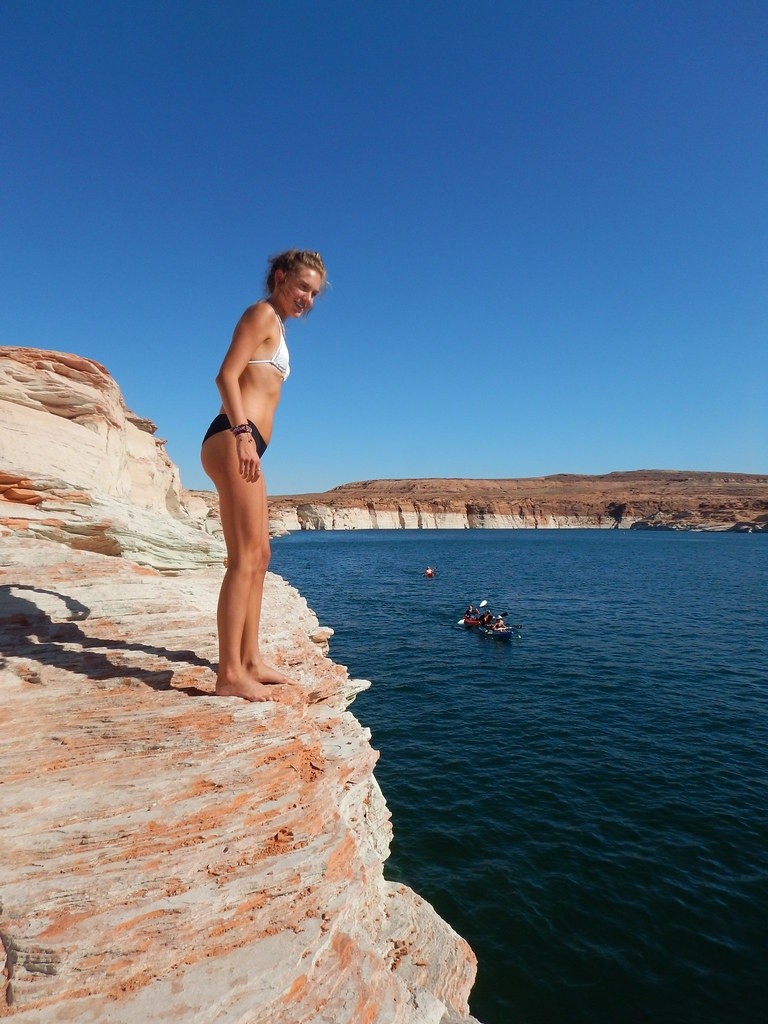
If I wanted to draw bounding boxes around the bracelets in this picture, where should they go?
[230,423,252,437]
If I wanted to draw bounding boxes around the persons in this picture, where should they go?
[464,605,480,620]
[426,566,433,574]
[490,615,506,630]
[196,247,328,703]
[478,609,495,628]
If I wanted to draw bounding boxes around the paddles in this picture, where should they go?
[501,612,509,617]
[457,599,488,625]
[422,566,439,577]
[495,625,522,631]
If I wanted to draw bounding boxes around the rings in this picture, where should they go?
[255,468,261,471]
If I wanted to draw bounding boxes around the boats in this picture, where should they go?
[456,617,513,640]
[422,572,437,577]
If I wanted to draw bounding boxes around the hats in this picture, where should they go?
[497,614,503,620]
